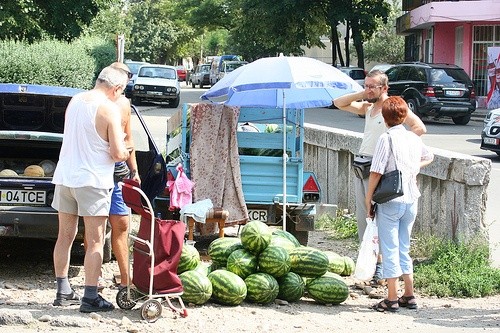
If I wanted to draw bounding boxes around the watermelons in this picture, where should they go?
[177,220,355,305]
[265,124,277,133]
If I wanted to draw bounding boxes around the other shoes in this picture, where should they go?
[96,277,107,289]
[118,284,124,290]
[370,261,388,288]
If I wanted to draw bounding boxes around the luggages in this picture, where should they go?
[117,181,188,323]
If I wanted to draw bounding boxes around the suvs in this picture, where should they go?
[323,66,367,109]
[123,61,152,98]
[356,61,477,126]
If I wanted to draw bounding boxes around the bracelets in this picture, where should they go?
[131,169,138,174]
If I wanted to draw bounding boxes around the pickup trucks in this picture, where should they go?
[131,64,181,109]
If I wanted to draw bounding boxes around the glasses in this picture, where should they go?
[364,84,384,89]
[120,68,133,78]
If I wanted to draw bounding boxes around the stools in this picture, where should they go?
[186,207,230,244]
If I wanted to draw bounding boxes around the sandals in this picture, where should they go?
[371,298,400,312]
[398,295,418,309]
[53,289,82,306]
[80,294,115,313]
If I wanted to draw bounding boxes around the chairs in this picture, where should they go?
[145,71,153,76]
[163,72,171,78]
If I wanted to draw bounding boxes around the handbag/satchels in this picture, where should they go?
[113,162,130,182]
[372,169,403,204]
[352,160,372,180]
[354,216,379,282]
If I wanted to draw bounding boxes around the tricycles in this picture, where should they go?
[164,101,322,247]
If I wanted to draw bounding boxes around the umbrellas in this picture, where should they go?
[200,52,365,232]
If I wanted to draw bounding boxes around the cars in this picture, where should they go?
[0,82,167,264]
[190,55,249,88]
[176,65,186,80]
[480,106,500,156]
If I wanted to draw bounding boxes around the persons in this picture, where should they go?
[365,95,434,313]
[50,65,133,312]
[333,70,427,287]
[487,62,500,109]
[185,57,193,85]
[85,61,141,293]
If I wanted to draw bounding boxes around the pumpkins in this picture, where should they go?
[0,169,18,177]
[24,165,45,177]
